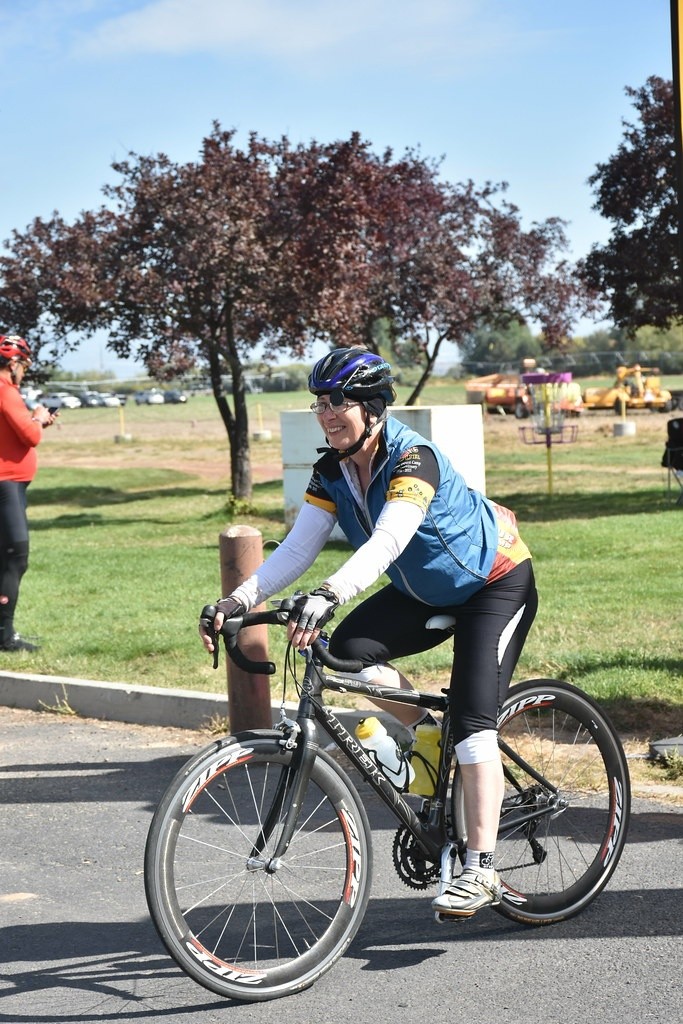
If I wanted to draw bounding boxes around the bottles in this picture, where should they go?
[408,717,441,796]
[354,717,416,788]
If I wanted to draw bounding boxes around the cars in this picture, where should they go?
[99,391,122,408]
[38,391,83,410]
[74,390,105,408]
[136,389,166,405]
[163,391,188,405]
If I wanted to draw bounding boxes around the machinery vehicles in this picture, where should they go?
[583,362,675,414]
[466,357,586,419]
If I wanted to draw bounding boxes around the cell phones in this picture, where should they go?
[48,407,59,415]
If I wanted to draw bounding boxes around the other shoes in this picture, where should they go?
[1,638,39,653]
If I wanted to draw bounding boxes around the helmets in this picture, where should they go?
[0,334,33,367]
[308,348,397,404]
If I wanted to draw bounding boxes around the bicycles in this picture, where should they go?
[142,588,632,1002]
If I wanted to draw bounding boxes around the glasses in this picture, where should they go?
[310,401,361,414]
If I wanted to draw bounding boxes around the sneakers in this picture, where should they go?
[431,869,502,916]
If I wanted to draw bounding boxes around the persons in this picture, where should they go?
[198,349,537,916]
[1,334,60,653]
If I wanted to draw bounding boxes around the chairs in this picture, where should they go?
[664,418,683,506]
